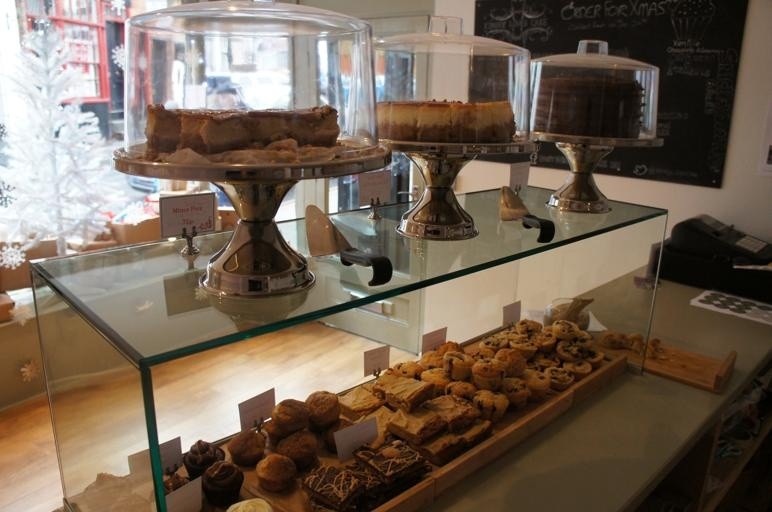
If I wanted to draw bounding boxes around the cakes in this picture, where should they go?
[509,340,538,361]
[256,452,296,492]
[470,357,505,392]
[532,75,645,141]
[270,398,311,437]
[541,324,553,336]
[182,439,226,482]
[434,341,465,368]
[466,347,495,362]
[544,367,575,391]
[501,330,529,341]
[582,349,605,369]
[388,406,448,445]
[142,101,342,156]
[406,430,468,466]
[324,418,354,455]
[571,329,594,350]
[228,427,267,468]
[261,419,284,451]
[376,99,518,144]
[530,332,557,353]
[552,319,579,340]
[421,350,437,370]
[562,360,593,382]
[442,351,475,381]
[338,386,383,422]
[478,334,509,353]
[202,459,243,508]
[302,461,365,511]
[372,374,435,414]
[362,405,396,449]
[305,390,342,428]
[351,438,427,485]
[494,347,527,377]
[520,368,551,402]
[515,318,542,335]
[393,360,423,380]
[526,360,540,371]
[275,429,319,470]
[455,418,493,448]
[420,394,481,433]
[420,367,451,398]
[226,497,273,512]
[444,380,478,400]
[472,389,509,423]
[533,351,561,371]
[556,340,582,362]
[500,376,529,412]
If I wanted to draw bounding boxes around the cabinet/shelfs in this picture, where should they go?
[26,185,669,512]
[0,265,772,512]
